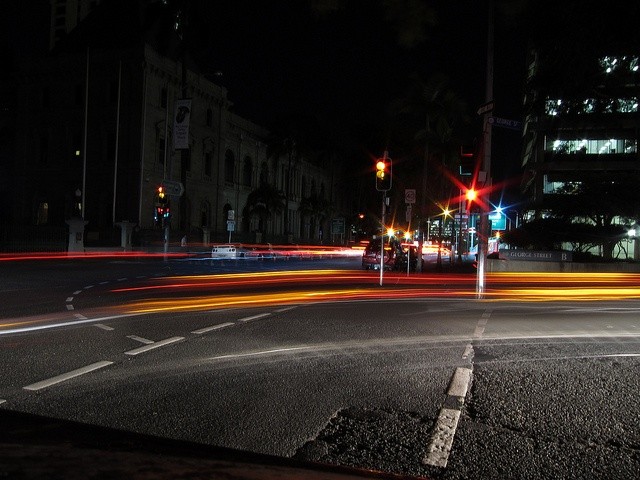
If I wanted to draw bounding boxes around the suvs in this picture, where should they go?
[362,238,424,271]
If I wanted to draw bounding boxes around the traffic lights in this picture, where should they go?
[376,158,392,191]
[157,206,170,218]
[159,186,167,203]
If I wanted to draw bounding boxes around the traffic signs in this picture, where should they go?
[488,116,521,131]
[476,101,495,115]
